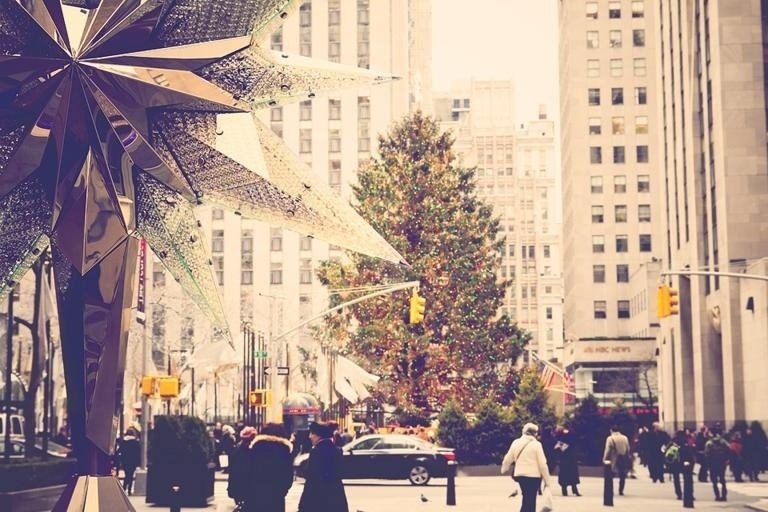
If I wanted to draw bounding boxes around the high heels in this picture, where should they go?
[573,491,581,496]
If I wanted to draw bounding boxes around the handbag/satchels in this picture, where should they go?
[612,455,632,473]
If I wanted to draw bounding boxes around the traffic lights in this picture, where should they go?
[142,376,155,396]
[408,295,429,326]
[656,285,682,318]
[248,390,264,405]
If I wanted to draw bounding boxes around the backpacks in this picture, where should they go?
[709,437,727,461]
[665,443,681,465]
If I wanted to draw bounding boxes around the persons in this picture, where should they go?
[602,424,632,495]
[501,422,555,512]
[356,411,430,439]
[114,410,353,512]
[557,437,581,496]
[637,420,768,508]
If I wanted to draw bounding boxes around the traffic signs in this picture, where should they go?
[251,351,268,358]
[263,366,291,376]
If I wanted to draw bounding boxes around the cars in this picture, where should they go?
[284,431,458,488]
[0,433,74,458]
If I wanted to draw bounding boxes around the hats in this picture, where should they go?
[239,426,257,440]
